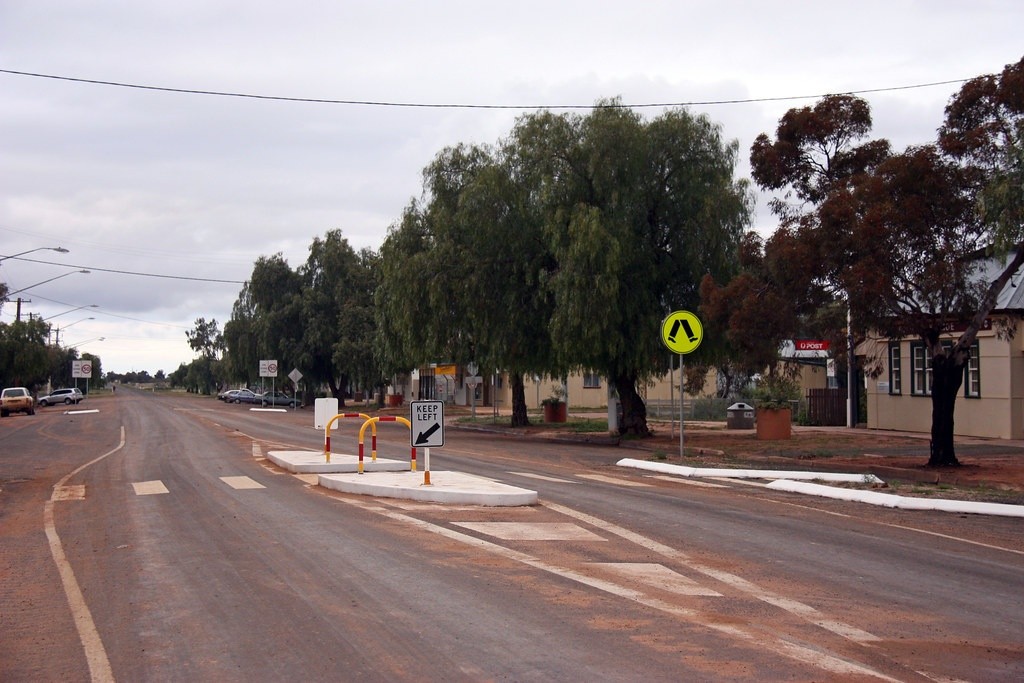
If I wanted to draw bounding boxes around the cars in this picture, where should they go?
[0,387,35,418]
[217,387,262,405]
[253,390,302,409]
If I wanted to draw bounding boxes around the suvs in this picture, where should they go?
[38,388,84,407]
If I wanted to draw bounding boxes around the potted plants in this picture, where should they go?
[388,387,405,406]
[738,377,796,441]
[541,386,569,422]
